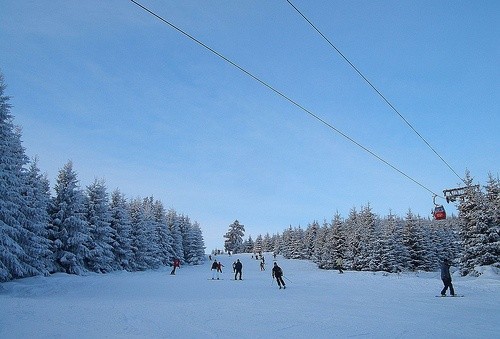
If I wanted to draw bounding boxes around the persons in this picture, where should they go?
[231,261,236,273]
[259,256,266,271]
[210,258,219,280]
[234,259,243,280]
[217,262,224,273]
[271,262,287,289]
[335,256,344,274]
[441,259,457,297]
[170,257,180,275]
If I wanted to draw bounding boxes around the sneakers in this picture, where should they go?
[279,287,281,288]
[217,278,219,279]
[239,278,241,280]
[235,279,237,279]
[284,285,285,287]
[441,291,446,295]
[451,293,456,296]
[212,278,214,279]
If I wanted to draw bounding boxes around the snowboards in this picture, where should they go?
[208,279,224,280]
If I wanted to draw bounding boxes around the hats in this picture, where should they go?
[215,259,216,261]
[274,262,276,264]
[237,259,239,261]
